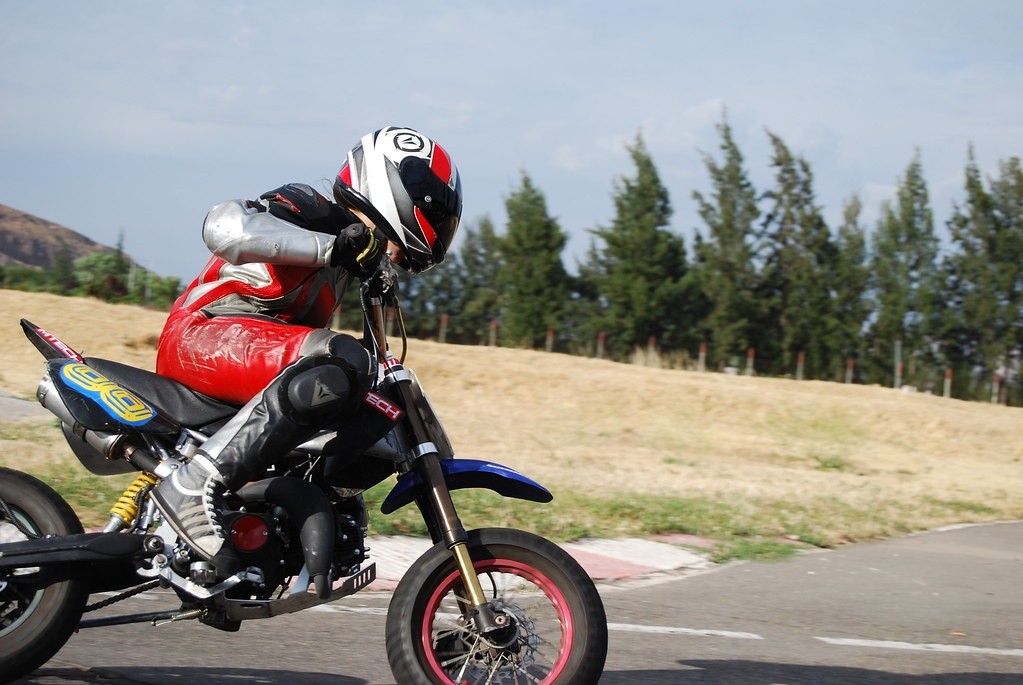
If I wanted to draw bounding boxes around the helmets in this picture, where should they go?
[333,126,464,273]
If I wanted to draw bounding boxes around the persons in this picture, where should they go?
[150,126,463,573]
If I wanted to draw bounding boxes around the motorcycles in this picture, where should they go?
[0,224,608,685]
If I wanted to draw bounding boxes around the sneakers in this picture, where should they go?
[148,456,250,574]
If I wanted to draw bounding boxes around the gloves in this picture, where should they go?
[333,223,388,270]
